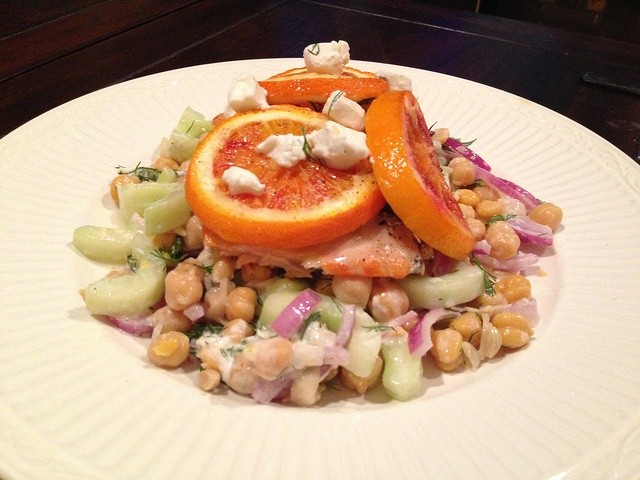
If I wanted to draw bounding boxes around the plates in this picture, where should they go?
[0,56,640,478]
